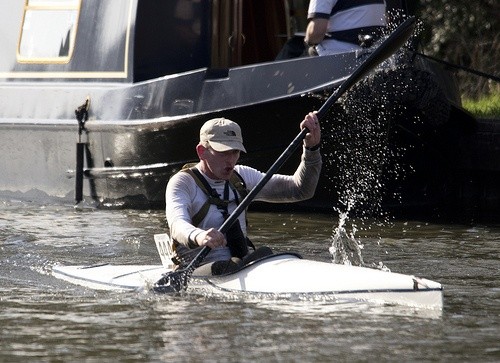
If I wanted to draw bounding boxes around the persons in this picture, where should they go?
[304,0,385,55]
[165,111,323,272]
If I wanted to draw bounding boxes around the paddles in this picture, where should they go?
[154,16,418,292]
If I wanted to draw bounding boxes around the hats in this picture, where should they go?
[199,118,247,154]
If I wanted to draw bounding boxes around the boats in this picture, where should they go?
[0,0,467,218]
[52,251,445,314]
